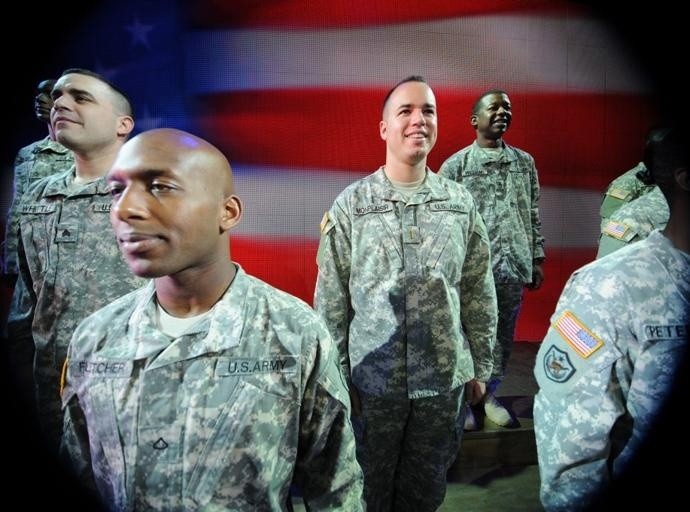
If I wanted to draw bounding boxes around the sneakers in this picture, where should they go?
[484,392,514,426]
[463,404,478,432]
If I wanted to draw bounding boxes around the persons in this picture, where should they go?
[313,73,499,511]
[20,66,155,511]
[1,80,76,418]
[64,127,367,511]
[436,89,547,431]
[533,102,689,512]
